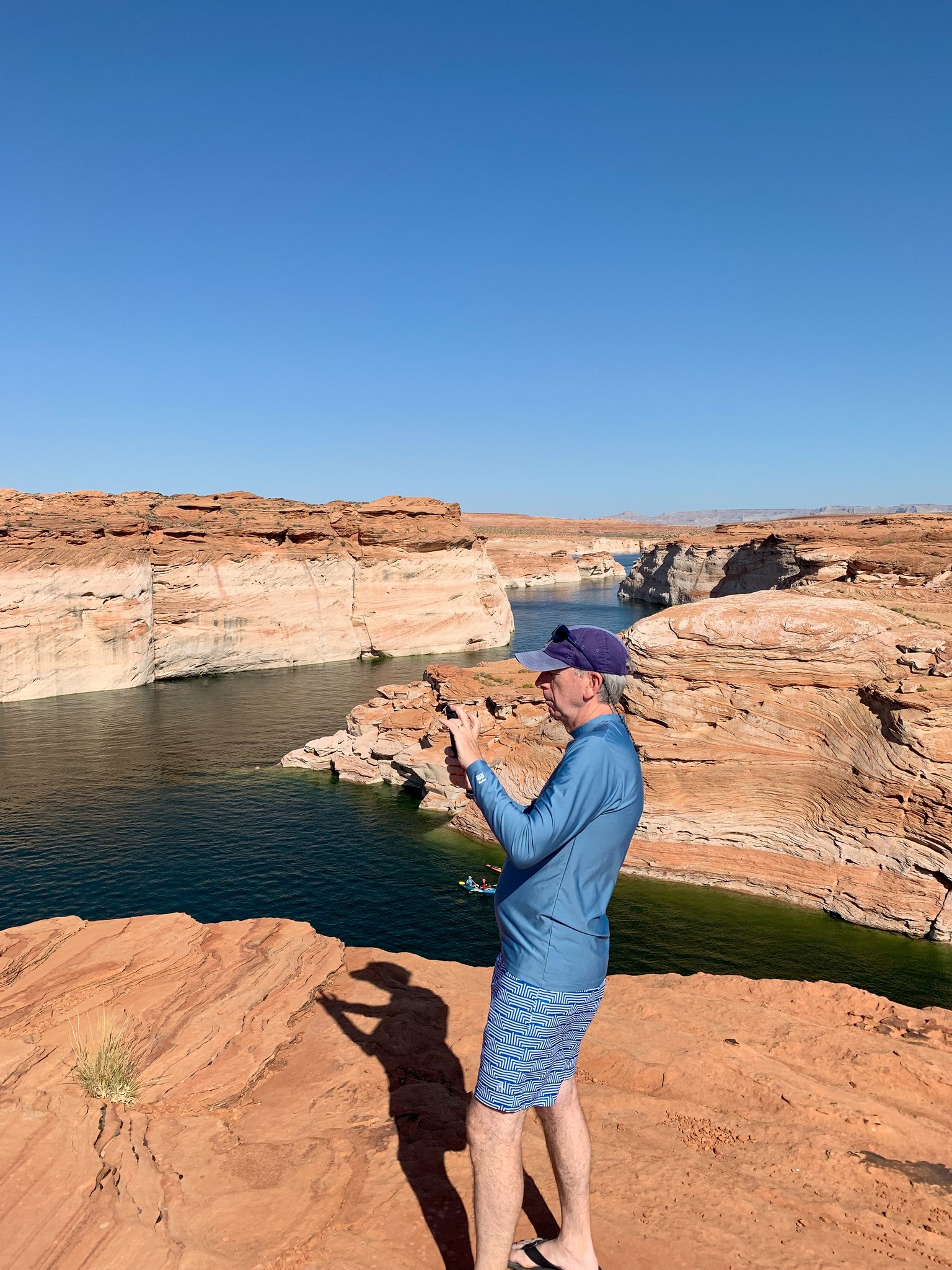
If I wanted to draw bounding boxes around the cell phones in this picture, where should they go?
[446,706,459,759]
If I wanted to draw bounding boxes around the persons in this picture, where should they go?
[438,623,644,1270]
[466,876,493,891]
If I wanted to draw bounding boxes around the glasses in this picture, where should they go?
[551,624,605,684]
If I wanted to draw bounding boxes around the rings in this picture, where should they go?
[458,724,463,728]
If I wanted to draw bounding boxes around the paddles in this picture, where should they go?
[470,884,497,891]
[459,881,477,884]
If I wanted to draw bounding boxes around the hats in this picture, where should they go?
[468,876,472,878]
[514,625,630,676]
[482,879,486,881]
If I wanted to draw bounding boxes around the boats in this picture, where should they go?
[459,881,496,894]
[486,864,502,873]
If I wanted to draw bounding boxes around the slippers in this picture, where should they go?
[507,1239,602,1270]
